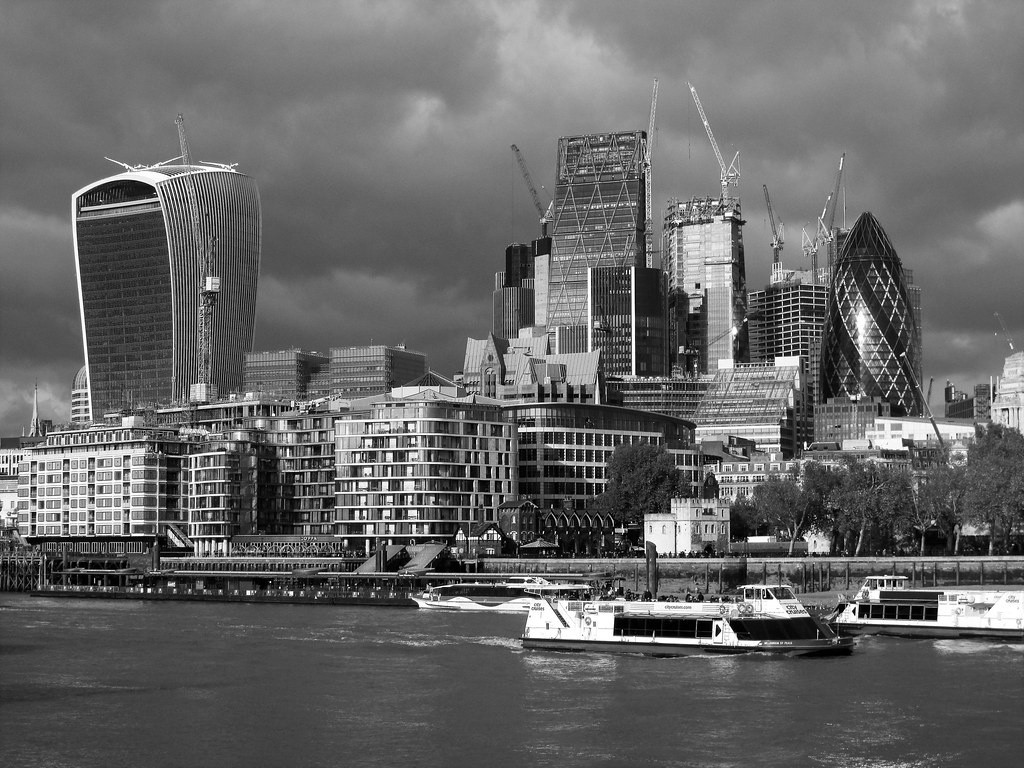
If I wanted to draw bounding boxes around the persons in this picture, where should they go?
[743,589,792,599]
[710,595,715,603]
[584,591,590,601]
[658,595,679,602]
[685,592,693,602]
[645,589,652,602]
[625,589,631,601]
[698,592,704,602]
[659,551,754,558]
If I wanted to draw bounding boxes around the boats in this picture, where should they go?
[519,583,856,659]
[28,568,421,609]
[828,576,1024,644]
[409,580,599,612]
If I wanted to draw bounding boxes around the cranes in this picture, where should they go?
[686,81,744,213]
[175,112,219,382]
[510,142,554,236]
[799,149,847,285]
[762,181,788,262]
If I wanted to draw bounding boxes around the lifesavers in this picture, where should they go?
[720,605,725,614]
[746,604,753,613]
[584,617,591,625]
[862,590,869,599]
[738,604,746,613]
